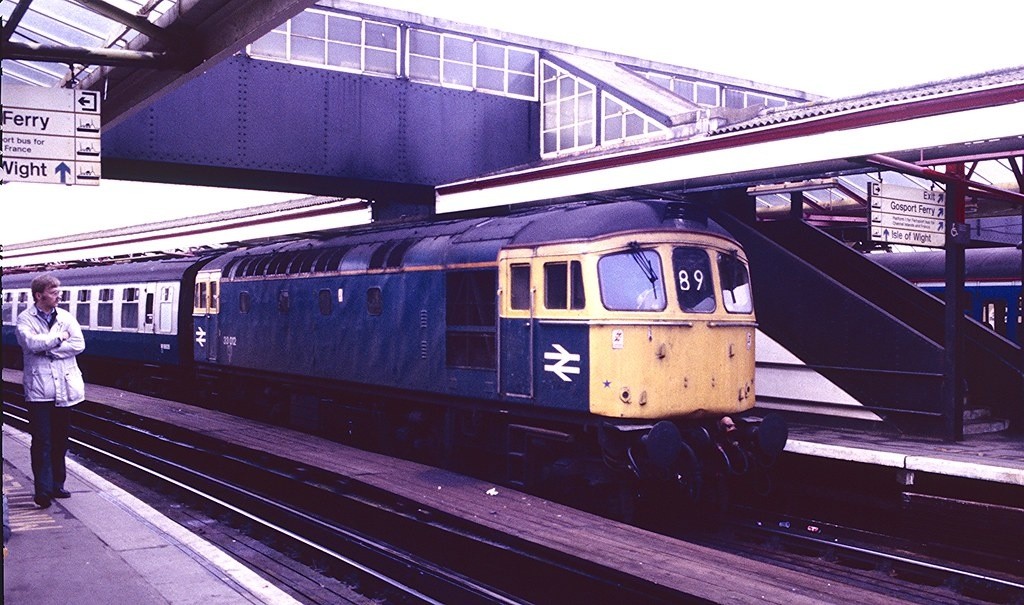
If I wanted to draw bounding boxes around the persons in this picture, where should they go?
[16,275,86,506]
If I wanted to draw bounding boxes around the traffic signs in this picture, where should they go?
[870,226,945,248]
[1,83,102,116]
[2,157,102,187]
[867,211,945,234]
[867,196,946,219]
[867,181,946,206]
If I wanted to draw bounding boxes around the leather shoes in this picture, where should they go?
[53,488,71,498]
[34,493,52,509]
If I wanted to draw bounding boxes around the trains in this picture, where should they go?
[859,250,1024,349]
[0,193,785,528]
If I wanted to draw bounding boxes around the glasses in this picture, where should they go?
[52,291,63,297]
